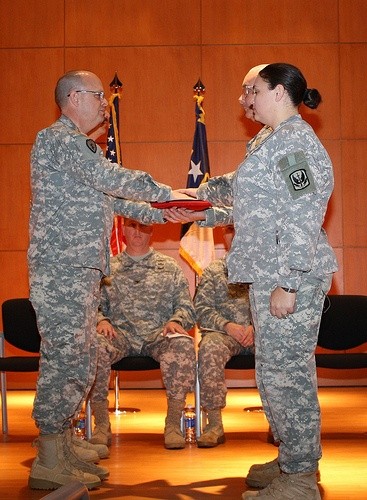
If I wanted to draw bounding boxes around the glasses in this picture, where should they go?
[67,90,103,100]
[243,85,253,95]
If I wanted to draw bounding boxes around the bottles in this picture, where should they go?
[74,413,86,439]
[183,404,198,444]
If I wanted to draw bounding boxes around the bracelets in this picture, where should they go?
[282,287,297,293]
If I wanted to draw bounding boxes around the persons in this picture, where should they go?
[163,63,340,500]
[27,68,198,490]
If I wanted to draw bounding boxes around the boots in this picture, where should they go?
[28,429,110,489]
[198,407,224,447]
[164,397,186,448]
[242,473,321,500]
[246,457,320,487]
[90,398,111,446]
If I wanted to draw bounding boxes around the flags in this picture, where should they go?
[179,78,215,279]
[104,73,127,258]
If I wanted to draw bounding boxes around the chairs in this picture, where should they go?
[0,293,367,439]
